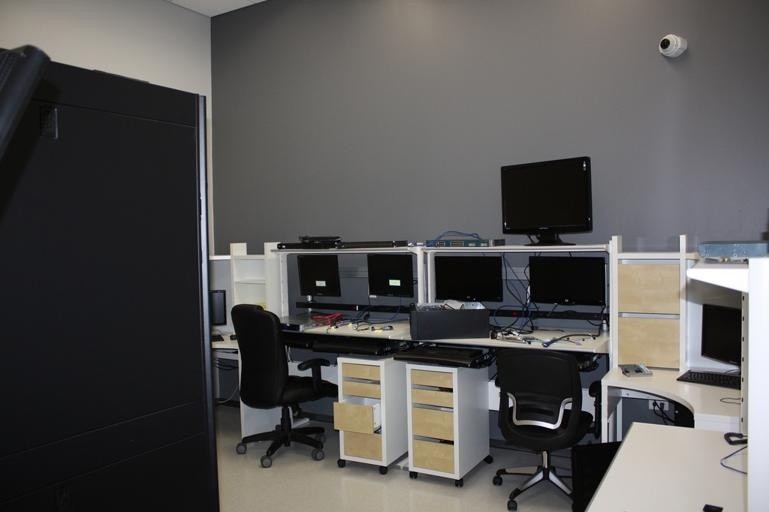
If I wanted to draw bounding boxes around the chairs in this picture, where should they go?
[231,303,338,469]
[494,350,593,512]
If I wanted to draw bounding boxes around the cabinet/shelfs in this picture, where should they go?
[333,354,408,474]
[406,360,493,488]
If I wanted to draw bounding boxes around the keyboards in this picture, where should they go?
[211,334,224,342]
[676,369,741,391]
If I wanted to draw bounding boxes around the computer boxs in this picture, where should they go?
[570,440,622,512]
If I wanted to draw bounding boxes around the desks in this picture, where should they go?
[211,332,242,400]
[305,320,608,355]
[583,364,748,512]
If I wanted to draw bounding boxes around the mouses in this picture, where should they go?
[230,334,236,340]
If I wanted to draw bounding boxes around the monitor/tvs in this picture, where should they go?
[435,255,504,303]
[367,253,415,299]
[701,303,742,368]
[297,254,342,298]
[209,289,227,326]
[529,255,605,307]
[501,156,594,235]
